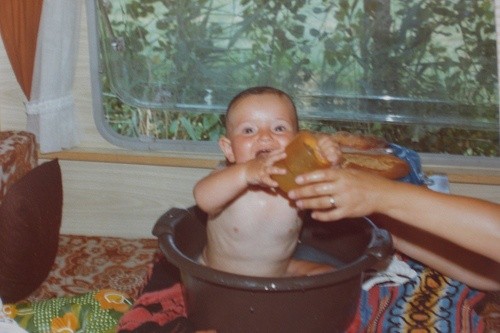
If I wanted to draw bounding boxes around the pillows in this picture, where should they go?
[0,157,63,303]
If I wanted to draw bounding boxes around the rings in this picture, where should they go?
[330,197,335,208]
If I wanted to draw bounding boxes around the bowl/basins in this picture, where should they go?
[152,203,393,333]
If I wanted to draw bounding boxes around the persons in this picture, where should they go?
[193,88,341,275]
[288,166,500,293]
